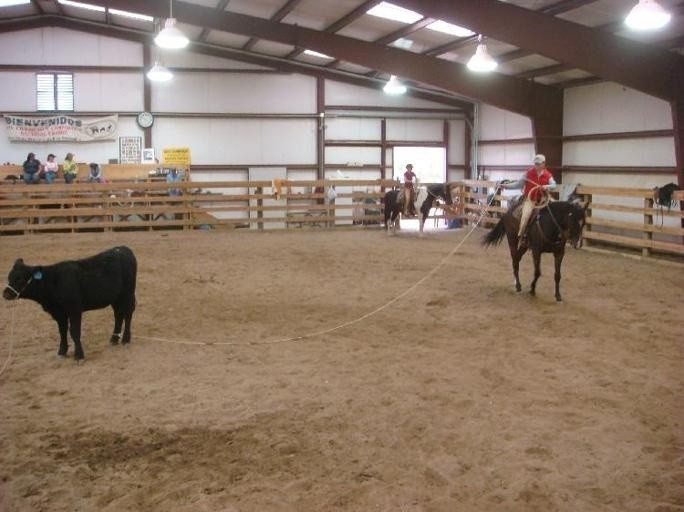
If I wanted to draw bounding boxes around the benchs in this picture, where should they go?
[1,162,193,183]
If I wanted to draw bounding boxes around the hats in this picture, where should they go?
[534,154,545,163]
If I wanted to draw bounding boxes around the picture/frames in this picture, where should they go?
[141,146,156,164]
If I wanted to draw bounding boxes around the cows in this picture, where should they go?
[2,245,138,366]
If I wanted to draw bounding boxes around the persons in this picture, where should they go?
[41,153,58,183]
[61,152,78,184]
[497,153,557,240]
[164,167,181,197]
[402,164,418,217]
[20,152,42,185]
[87,163,103,183]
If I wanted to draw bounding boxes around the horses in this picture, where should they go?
[383,182,454,237]
[478,200,591,305]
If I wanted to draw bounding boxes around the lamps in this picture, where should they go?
[150,0,191,51]
[382,72,408,97]
[465,32,499,74]
[144,47,175,84]
[623,0,673,34]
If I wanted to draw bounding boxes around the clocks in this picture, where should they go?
[135,110,155,129]
